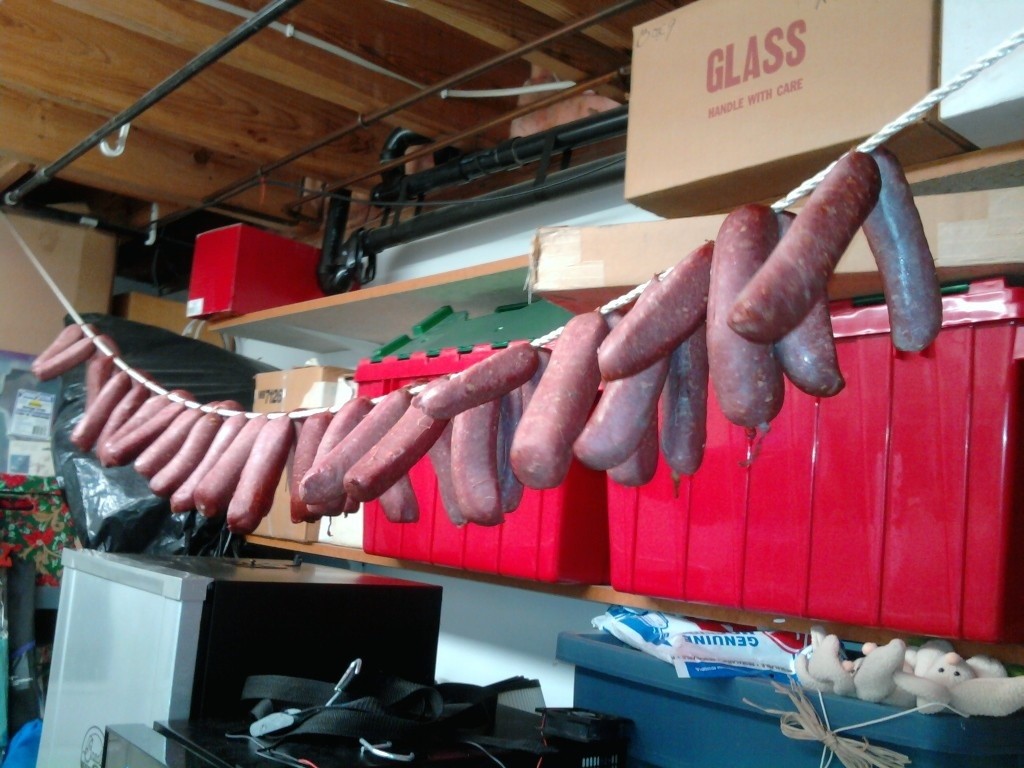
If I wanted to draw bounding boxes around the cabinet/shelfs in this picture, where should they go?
[207,137,1024,671]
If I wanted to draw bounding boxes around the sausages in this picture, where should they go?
[30,321,295,534]
[286,145,940,529]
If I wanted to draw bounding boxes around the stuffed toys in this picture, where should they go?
[797,625,1024,719]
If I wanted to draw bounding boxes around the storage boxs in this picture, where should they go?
[597,273,1024,647]
[321,374,364,550]
[0,207,117,355]
[247,363,355,544]
[111,290,228,348]
[941,1,1024,149]
[621,0,978,222]
[553,629,1024,768]
[523,186,1024,314]
[352,296,611,587]
[183,223,364,326]
